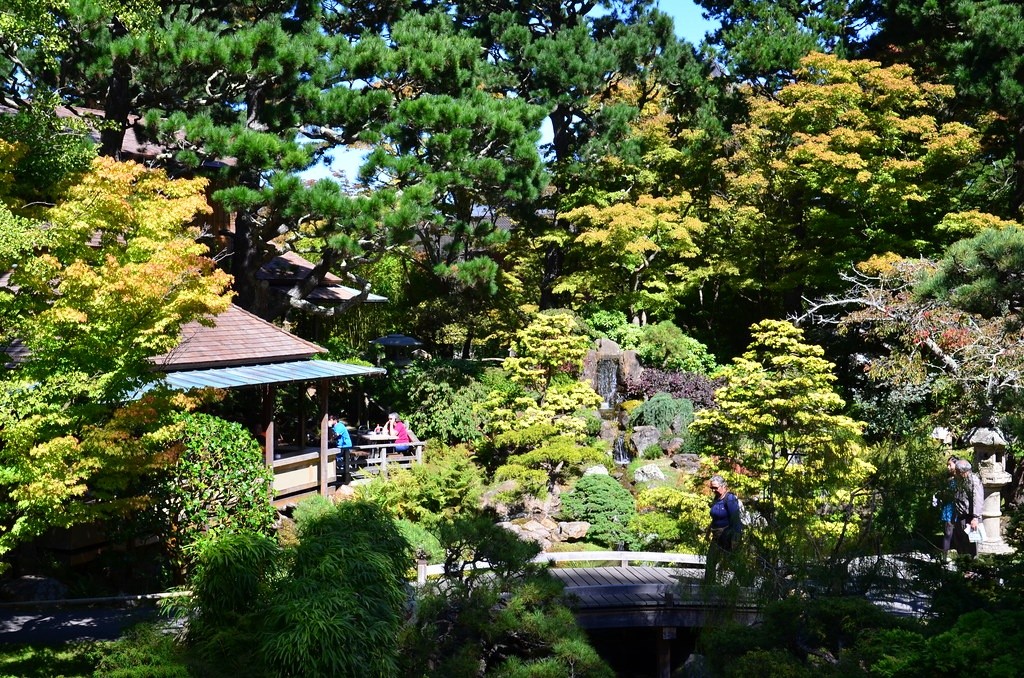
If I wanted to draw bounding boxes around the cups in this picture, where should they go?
[380,428,382,434]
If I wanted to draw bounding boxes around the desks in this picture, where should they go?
[356,433,398,467]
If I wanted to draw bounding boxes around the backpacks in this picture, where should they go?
[723,492,752,529]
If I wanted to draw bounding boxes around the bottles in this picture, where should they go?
[274,448,281,460]
[376,424,380,434]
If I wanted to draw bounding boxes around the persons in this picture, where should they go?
[251,423,266,460]
[382,412,409,458]
[328,415,352,469]
[704,476,755,587]
[941,456,983,579]
